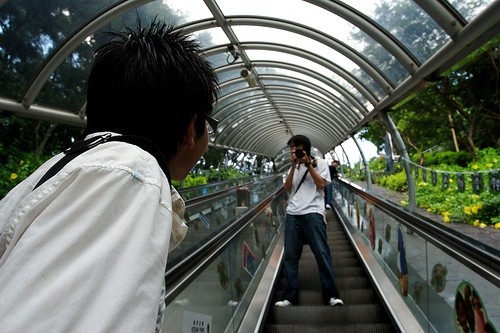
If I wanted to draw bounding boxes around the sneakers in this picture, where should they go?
[330,298,344,305]
[275,300,292,307]
[326,204,331,209]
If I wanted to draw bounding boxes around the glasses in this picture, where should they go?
[205,116,219,134]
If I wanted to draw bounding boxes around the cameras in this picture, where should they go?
[293,149,305,158]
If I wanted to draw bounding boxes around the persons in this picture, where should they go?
[237,183,251,206]
[0,15,221,333]
[274,135,344,307]
[324,160,340,209]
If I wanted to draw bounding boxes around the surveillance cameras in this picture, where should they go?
[228,44,241,54]
